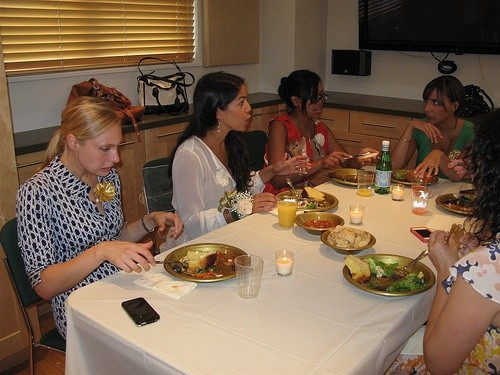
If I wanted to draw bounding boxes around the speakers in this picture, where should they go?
[331,49,373,76]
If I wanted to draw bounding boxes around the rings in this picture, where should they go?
[295,166,298,169]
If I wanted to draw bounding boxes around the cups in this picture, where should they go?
[391,183,405,201]
[275,250,294,277]
[276,191,298,228]
[234,254,264,299]
[356,170,375,196]
[350,201,365,225]
[410,173,433,215]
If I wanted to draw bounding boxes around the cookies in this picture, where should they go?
[449,151,462,162]
[446,224,466,249]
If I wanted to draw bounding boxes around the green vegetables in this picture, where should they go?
[306,202,316,209]
[363,256,427,292]
[451,196,472,207]
[395,171,408,180]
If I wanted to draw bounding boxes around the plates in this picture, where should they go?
[329,168,357,185]
[164,243,249,283]
[297,190,338,212]
[435,193,477,215]
[389,168,438,186]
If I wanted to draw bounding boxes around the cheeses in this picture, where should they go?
[181,250,217,267]
[304,187,326,201]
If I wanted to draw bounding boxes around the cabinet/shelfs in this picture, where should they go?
[12,93,424,254]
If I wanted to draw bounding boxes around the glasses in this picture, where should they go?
[298,93,329,104]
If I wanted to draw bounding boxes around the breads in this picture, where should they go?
[406,171,433,183]
[357,152,381,162]
[344,254,370,281]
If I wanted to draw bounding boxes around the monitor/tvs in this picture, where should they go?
[358,0,500,55]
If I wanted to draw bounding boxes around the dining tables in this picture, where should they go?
[65,165,476,375]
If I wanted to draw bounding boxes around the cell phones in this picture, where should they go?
[120,297,160,328]
[411,227,436,244]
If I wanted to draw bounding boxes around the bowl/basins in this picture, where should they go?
[294,211,345,235]
[342,254,436,296]
[321,229,376,254]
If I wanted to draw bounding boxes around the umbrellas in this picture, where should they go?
[67,78,143,143]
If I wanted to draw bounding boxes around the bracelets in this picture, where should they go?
[400,137,412,145]
[141,215,154,233]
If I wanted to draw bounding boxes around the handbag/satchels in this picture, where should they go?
[137,57,195,116]
[455,84,494,118]
[65,77,145,143]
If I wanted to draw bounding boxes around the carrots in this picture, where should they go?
[193,253,233,279]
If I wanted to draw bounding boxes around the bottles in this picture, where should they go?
[374,140,391,194]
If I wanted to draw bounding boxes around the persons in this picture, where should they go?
[262,69,381,198]
[390,75,480,183]
[15,96,184,343]
[384,106,500,375]
[158,72,312,255]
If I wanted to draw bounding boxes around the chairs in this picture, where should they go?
[225,130,267,171]
[142,157,176,256]
[0,218,66,375]
